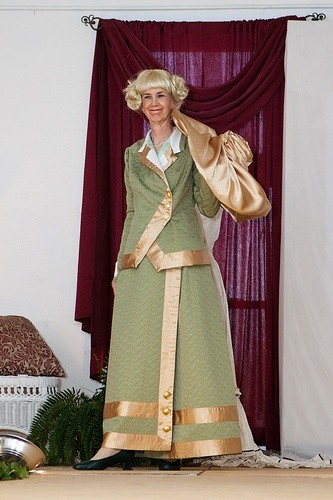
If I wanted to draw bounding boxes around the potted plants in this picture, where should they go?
[26,365,108,466]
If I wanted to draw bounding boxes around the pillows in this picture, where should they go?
[0,315,66,376]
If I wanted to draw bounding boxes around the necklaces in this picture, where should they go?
[153,142,163,148]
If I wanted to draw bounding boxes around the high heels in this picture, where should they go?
[72,447,134,471]
[151,457,181,470]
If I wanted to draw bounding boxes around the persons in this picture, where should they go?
[72,69,243,471]
